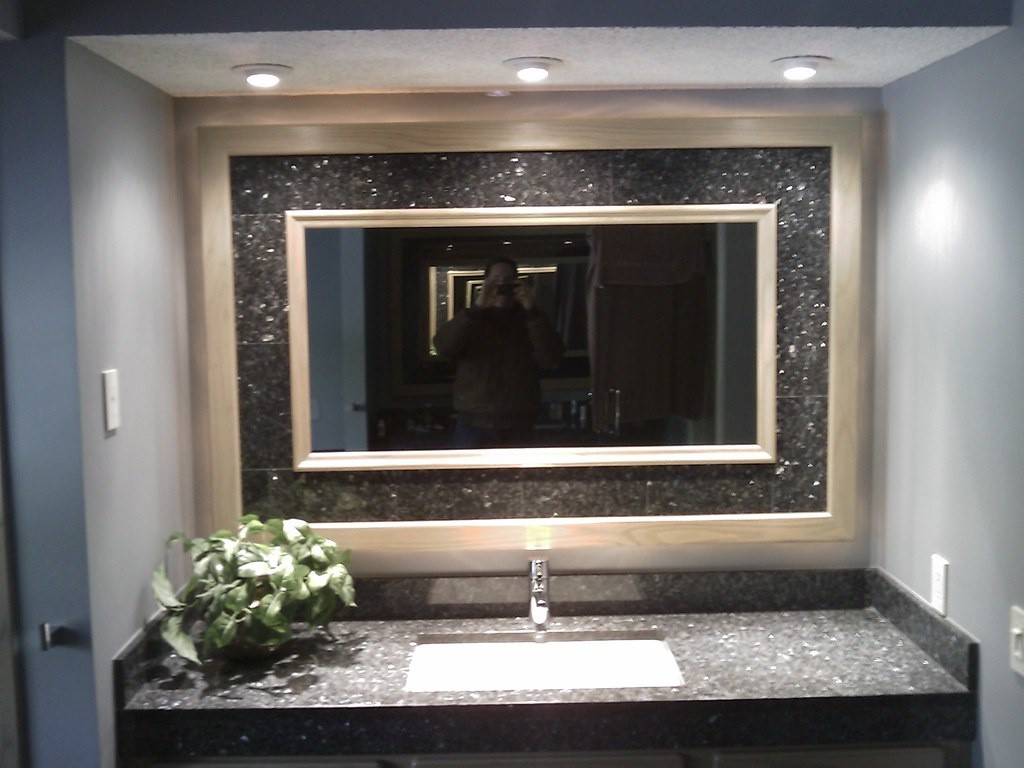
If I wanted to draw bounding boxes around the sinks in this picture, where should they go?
[403,628,685,691]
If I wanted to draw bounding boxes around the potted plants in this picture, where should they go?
[146,511,357,667]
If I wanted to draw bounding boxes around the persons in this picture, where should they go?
[432,258,552,450]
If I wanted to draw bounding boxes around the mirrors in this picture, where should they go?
[284,203,780,474]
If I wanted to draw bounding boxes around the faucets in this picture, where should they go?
[528,559,551,631]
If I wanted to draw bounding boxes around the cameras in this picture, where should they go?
[497,283,520,295]
